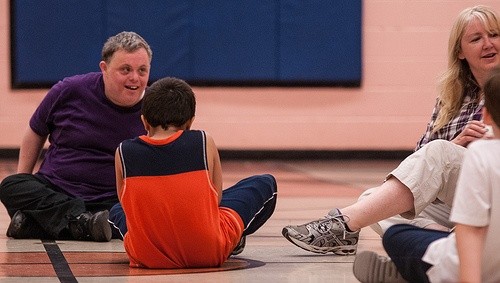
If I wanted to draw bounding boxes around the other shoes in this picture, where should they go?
[353,251,404,283]
[6,211,46,237]
[69,210,113,242]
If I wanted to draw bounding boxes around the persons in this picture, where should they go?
[108,77,278,269]
[282,5,500,256]
[352,73,500,283]
[0,31,153,243]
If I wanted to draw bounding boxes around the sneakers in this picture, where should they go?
[281,208,360,256]
